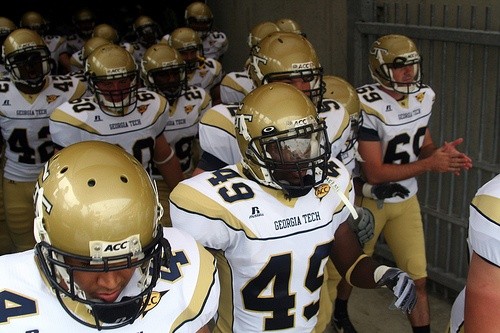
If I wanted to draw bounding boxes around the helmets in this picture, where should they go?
[1,29,50,93]
[171,28,201,72]
[249,21,281,50]
[235,83,319,183]
[248,33,322,110]
[85,39,113,58]
[319,75,361,127]
[91,24,119,44]
[134,16,152,37]
[276,18,306,37]
[185,3,215,33]
[33,142,159,271]
[24,12,42,29]
[0,17,17,40]
[368,33,420,81]
[85,45,137,93]
[141,44,187,97]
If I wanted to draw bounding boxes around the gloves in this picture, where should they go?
[373,265,417,314]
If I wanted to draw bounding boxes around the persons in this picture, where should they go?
[0,0,410,256]
[330,35,472,333]
[168,82,417,333]
[0,141,220,333]
[445,173,500,333]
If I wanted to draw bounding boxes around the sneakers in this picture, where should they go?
[331,314,357,333]
